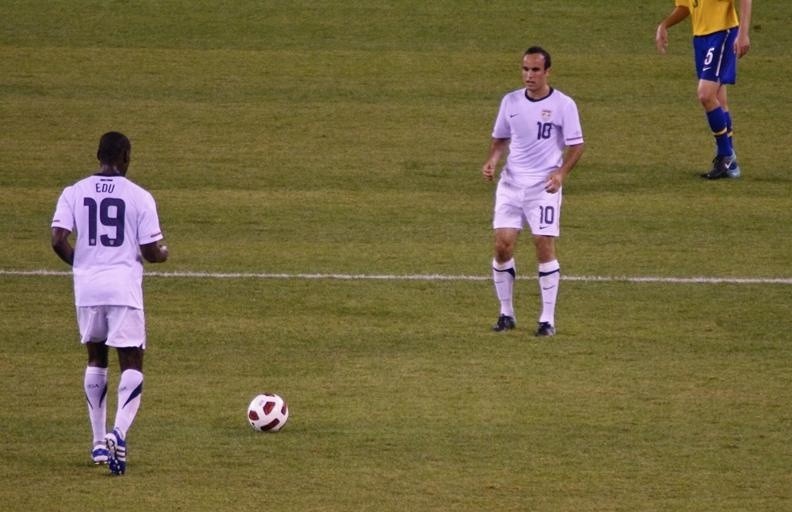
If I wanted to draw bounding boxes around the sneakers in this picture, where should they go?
[91,440,108,465]
[535,321,556,337]
[494,314,516,332]
[701,150,740,179]
[105,426,127,475]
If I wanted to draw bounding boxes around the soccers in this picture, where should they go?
[248,394,288,433]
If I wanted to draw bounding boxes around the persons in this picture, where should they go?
[482,45,585,340]
[50,131,169,476]
[654,1,755,180]
